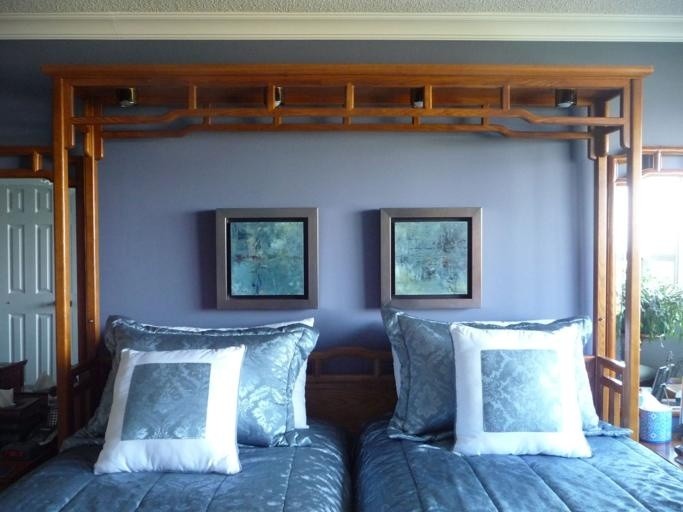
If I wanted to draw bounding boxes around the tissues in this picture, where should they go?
[638,388,675,444]
[0,387,43,435]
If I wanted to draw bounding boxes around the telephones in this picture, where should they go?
[39,391,60,433]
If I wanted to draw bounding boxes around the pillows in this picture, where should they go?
[90,315,318,452]
[381,312,597,458]
[92,344,248,474]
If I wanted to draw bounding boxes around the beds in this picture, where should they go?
[355,409,681,510]
[7,410,351,510]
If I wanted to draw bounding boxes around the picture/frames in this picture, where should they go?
[217,208,318,309]
[380,207,482,310]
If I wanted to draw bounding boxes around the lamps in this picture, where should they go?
[117,87,577,110]
